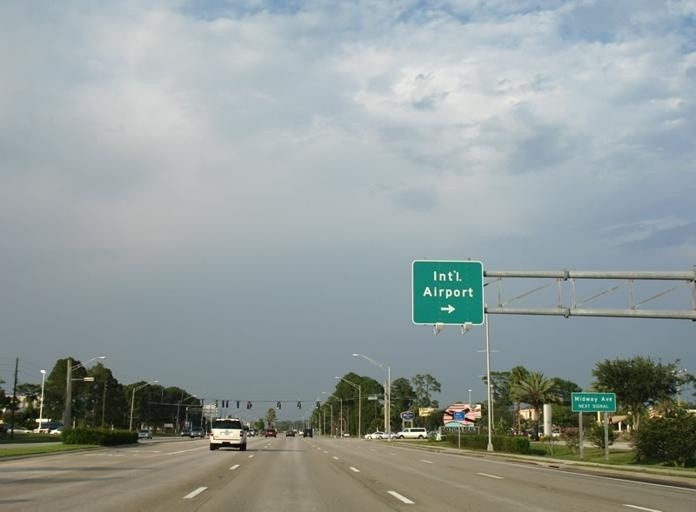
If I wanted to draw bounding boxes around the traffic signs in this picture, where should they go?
[412,260,484,326]
[571,392,617,412]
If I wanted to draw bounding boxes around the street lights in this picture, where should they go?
[175,395,198,433]
[467,388,472,404]
[64,356,108,427]
[310,354,390,438]
[130,381,159,431]
[38,369,46,429]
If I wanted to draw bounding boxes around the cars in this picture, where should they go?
[181,418,312,451]
[365,428,439,440]
[6,426,64,435]
[138,430,152,439]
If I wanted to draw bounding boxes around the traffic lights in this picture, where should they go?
[216,400,320,409]
